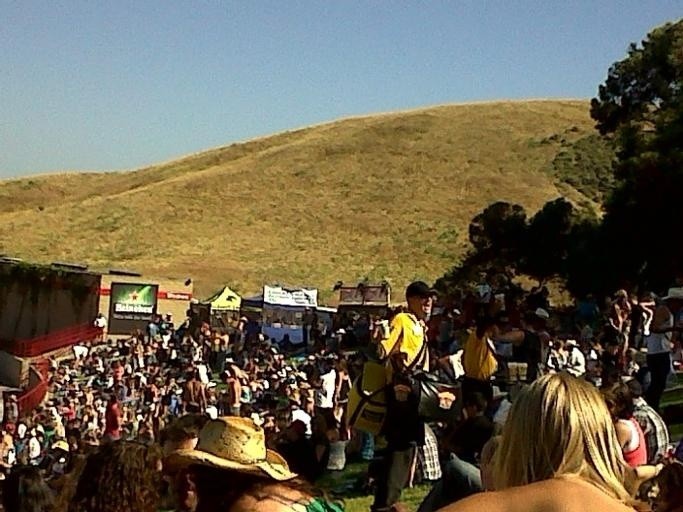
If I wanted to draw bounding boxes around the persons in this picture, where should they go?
[418,389,512,511]
[441,374,637,512]
[372,281,439,512]
[425,271,682,413]
[0,314,366,510]
[602,377,683,512]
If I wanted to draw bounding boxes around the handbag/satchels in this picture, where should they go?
[344,360,388,437]
[415,378,464,424]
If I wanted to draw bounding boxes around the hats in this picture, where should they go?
[405,280,440,298]
[563,339,581,348]
[168,414,300,483]
